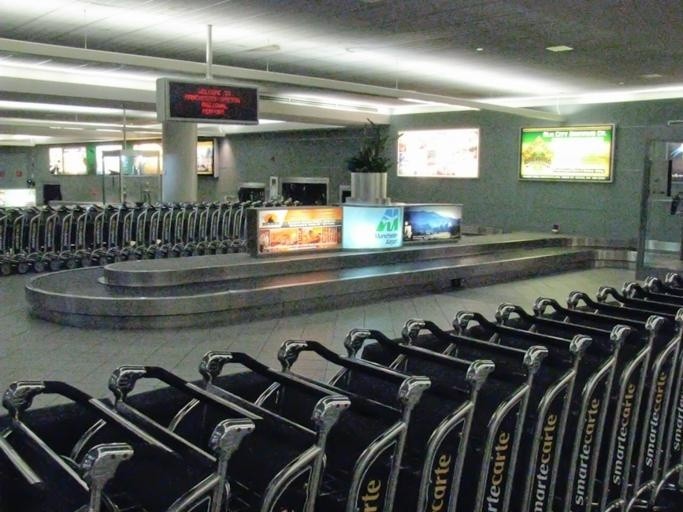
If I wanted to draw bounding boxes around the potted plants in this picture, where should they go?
[343,118,400,198]
